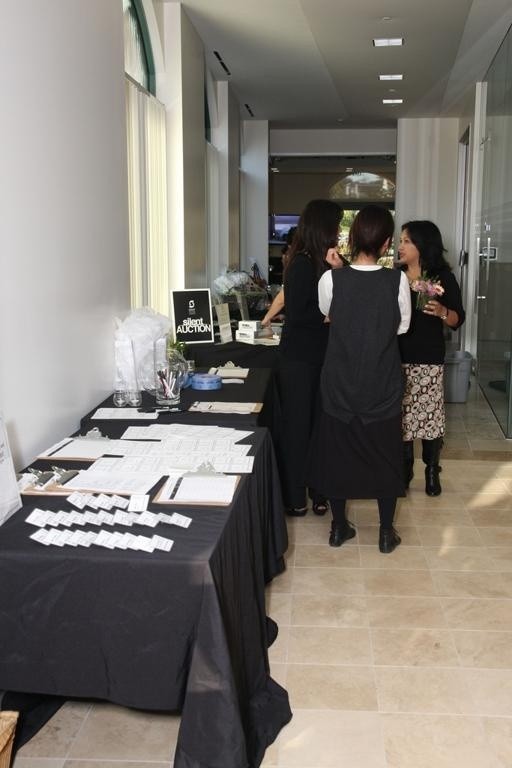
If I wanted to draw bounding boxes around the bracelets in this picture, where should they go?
[441,307,449,320]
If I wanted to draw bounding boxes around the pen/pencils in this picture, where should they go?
[157,368,176,399]
[48,440,75,457]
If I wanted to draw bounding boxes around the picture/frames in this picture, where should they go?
[172,287,215,345]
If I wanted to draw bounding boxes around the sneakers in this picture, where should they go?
[287,507,307,516]
[313,501,329,515]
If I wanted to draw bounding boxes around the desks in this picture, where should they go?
[0,427,293,768]
[78,315,290,516]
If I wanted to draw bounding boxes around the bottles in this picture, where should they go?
[154,335,181,407]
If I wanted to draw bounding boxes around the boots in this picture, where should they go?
[379,526,401,553]
[328,521,356,547]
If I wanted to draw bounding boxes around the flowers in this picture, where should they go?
[411,274,447,313]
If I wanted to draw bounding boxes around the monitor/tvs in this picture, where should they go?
[272,212,301,237]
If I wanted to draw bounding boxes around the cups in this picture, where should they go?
[112,338,143,407]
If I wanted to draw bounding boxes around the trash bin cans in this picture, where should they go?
[444,351,473,403]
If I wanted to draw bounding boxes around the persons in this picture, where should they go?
[273,199,349,515]
[395,220,465,499]
[257,207,306,332]
[315,204,414,553]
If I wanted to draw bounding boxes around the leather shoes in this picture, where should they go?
[425,466,442,495]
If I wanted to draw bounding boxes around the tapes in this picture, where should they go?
[192,373,222,390]
